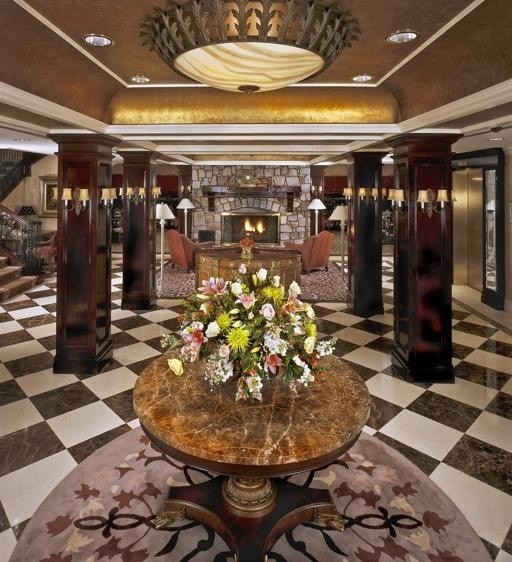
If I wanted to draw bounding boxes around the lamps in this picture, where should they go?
[17,206,36,223]
[306,197,326,239]
[155,197,195,296]
[138,0,363,95]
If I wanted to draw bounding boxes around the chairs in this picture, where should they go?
[283,231,334,273]
[25,231,58,273]
[164,229,214,273]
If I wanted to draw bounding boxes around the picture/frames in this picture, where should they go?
[38,175,58,218]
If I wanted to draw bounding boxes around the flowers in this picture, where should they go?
[158,237,338,402]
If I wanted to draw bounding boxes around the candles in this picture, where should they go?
[62,187,161,201]
[342,188,448,202]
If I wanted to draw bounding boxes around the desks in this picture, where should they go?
[133,352,370,560]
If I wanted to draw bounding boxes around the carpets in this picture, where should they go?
[6,426,495,561]
[157,261,350,302]
